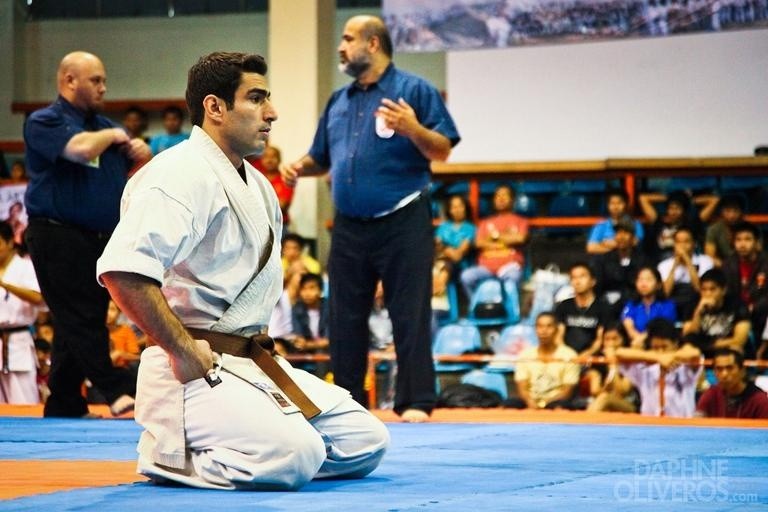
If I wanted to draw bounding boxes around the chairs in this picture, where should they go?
[292,242,531,398]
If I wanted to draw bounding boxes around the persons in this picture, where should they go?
[284,15,463,421]
[98,52,392,492]
[148,104,190,155]
[23,49,153,418]
[120,105,151,144]
[0,147,768,418]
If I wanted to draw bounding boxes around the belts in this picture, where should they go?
[23,215,111,244]
[332,186,435,228]
